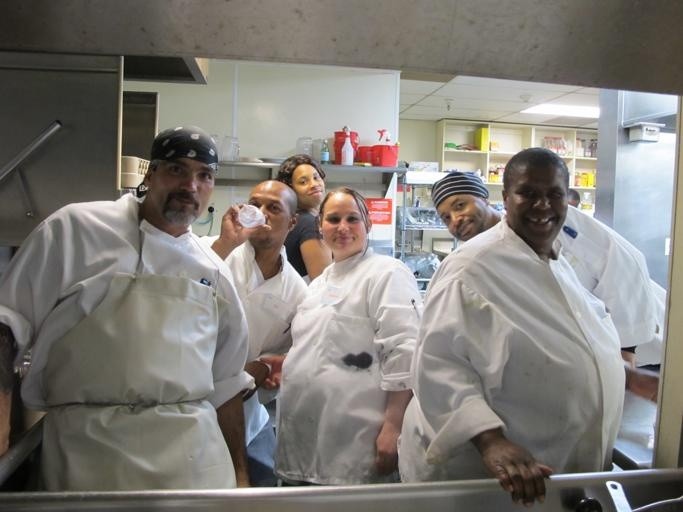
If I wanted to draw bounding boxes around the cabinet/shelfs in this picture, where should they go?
[395,118,597,293]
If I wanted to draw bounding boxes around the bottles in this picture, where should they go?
[237,204,266,229]
[574,171,588,187]
[319,139,331,165]
[576,137,598,159]
[542,135,567,155]
[489,163,505,182]
[476,168,482,177]
[580,192,592,217]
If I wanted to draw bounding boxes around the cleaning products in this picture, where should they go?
[341,126,353,165]
[377,129,391,144]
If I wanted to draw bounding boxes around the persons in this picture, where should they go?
[395,145,627,507]
[259,185,422,488]
[277,153,334,287]
[197,178,310,488]
[429,169,668,373]
[1,122,256,493]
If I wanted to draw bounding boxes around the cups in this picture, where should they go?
[121,155,150,188]
[212,134,241,162]
[302,136,313,155]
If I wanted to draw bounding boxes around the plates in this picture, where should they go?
[258,157,287,164]
